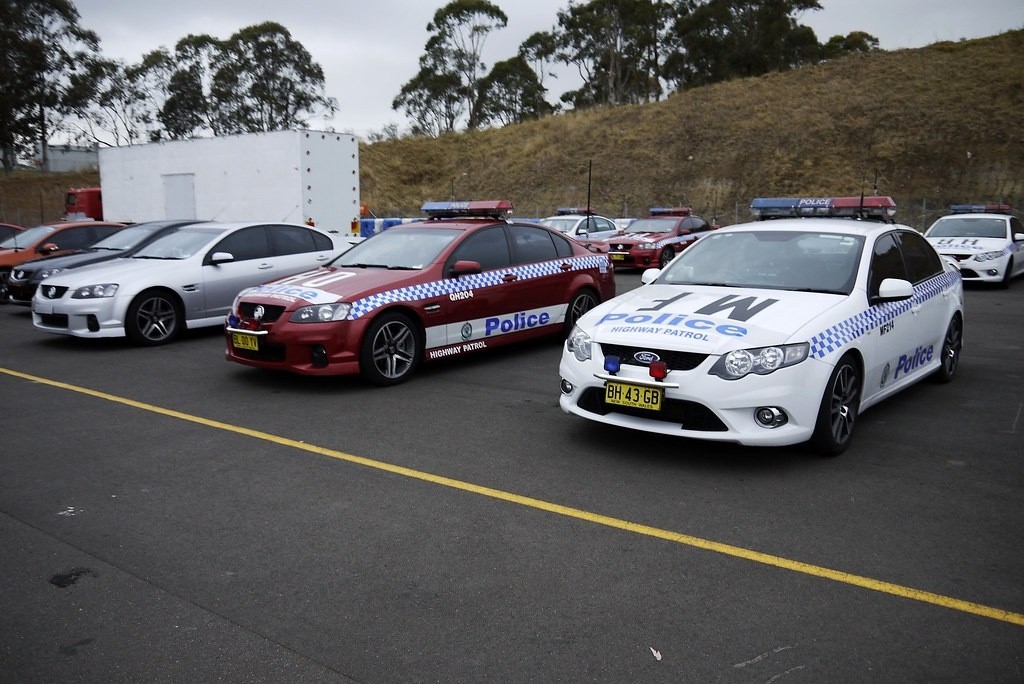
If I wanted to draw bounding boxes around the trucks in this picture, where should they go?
[67,130,361,246]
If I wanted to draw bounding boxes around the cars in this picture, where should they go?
[508,205,721,272]
[0,219,306,308]
[223,198,618,391]
[923,203,1024,292]
[555,193,967,459]
[29,219,359,349]
